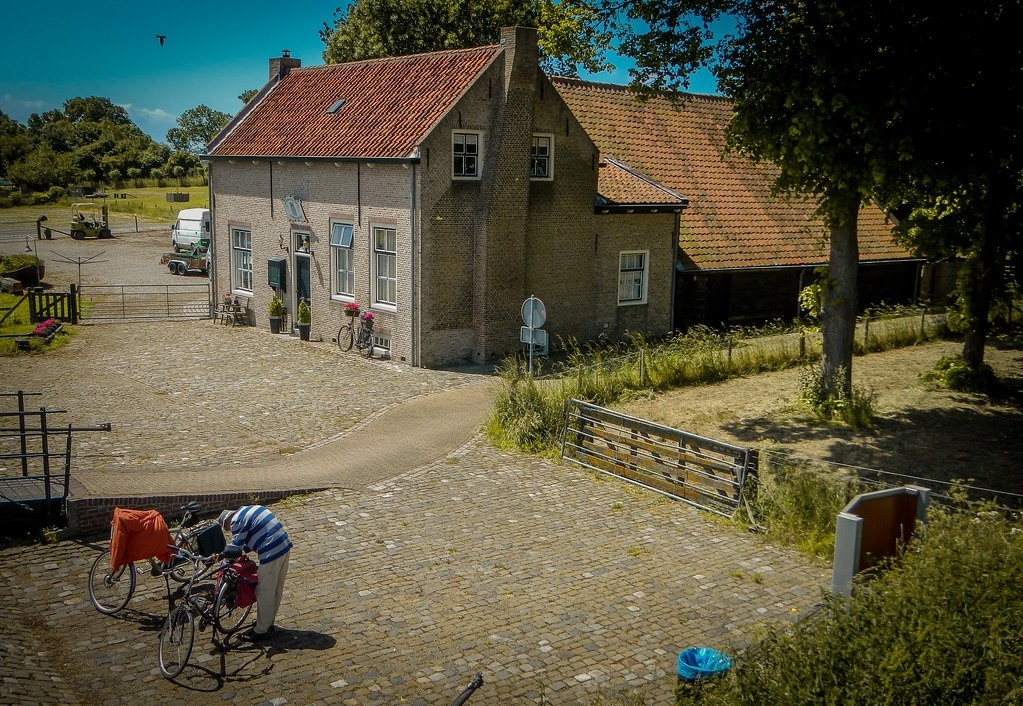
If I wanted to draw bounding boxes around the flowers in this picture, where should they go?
[344,303,359,310]
[364,313,375,320]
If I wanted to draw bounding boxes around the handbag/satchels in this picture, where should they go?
[197,524,227,567]
[216,559,259,608]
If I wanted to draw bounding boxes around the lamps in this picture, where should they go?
[278,234,289,253]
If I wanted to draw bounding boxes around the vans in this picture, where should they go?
[170,208,210,255]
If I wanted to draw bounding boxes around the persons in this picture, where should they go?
[212,505,294,639]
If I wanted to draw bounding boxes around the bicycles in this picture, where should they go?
[337,304,375,359]
[157,544,257,679]
[89,501,227,613]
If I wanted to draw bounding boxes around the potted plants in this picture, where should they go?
[296,299,312,341]
[267,294,283,333]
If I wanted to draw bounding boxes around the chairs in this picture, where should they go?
[213,294,253,328]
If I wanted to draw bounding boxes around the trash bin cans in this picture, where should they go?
[676,644,732,703]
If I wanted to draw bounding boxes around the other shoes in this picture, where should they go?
[252,619,274,630]
[241,628,272,642]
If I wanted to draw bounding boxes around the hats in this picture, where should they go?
[218,510,236,532]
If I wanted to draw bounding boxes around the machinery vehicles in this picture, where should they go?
[70,201,112,241]
